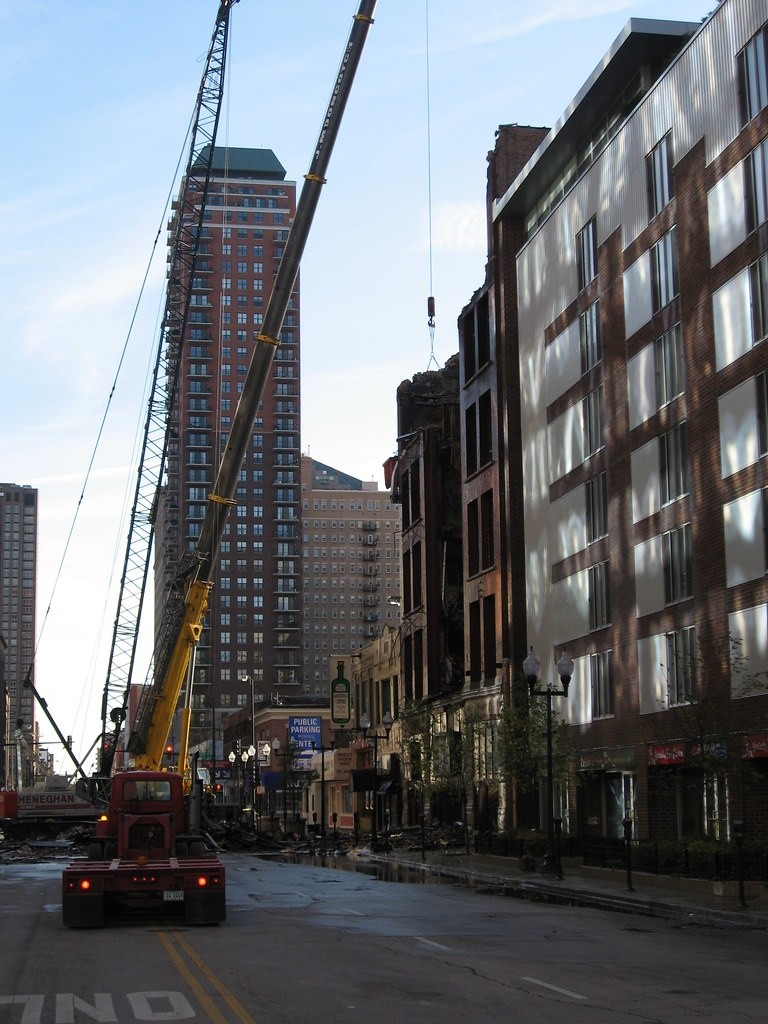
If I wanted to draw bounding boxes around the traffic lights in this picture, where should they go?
[215,784,222,790]
[167,746,171,759]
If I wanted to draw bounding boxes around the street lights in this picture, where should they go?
[522,643,575,869]
[271,735,295,821]
[309,735,335,836]
[359,710,394,841]
[247,743,271,817]
[227,750,248,803]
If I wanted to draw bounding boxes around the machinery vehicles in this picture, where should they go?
[59,0,437,925]
[0,0,240,838]
